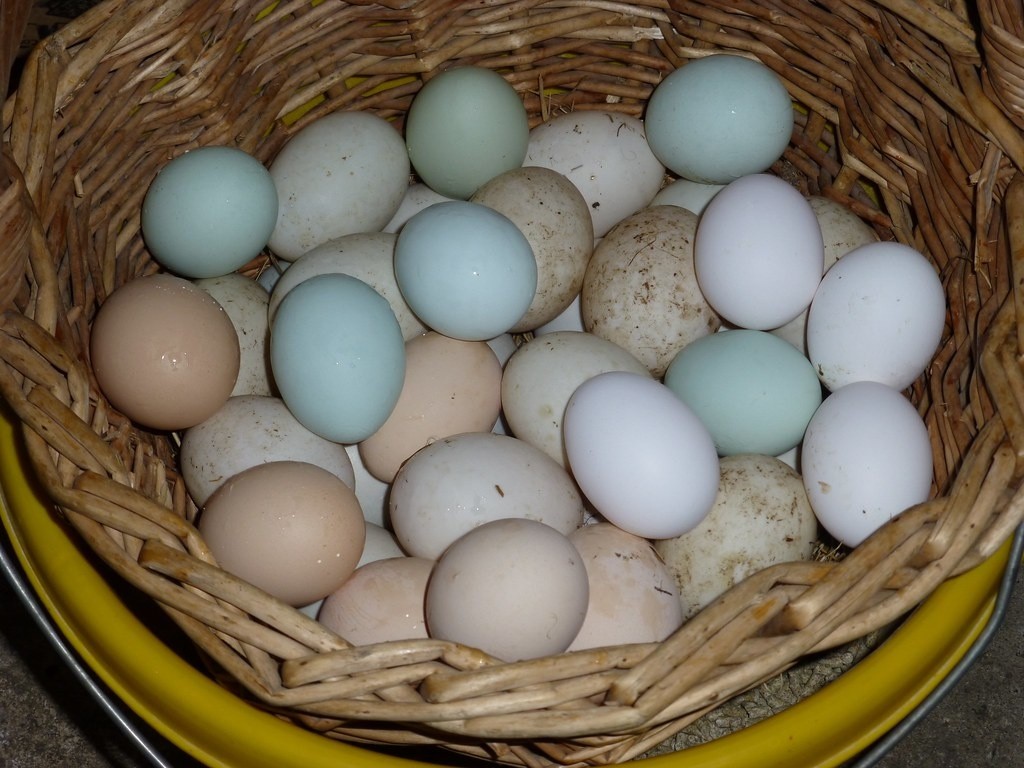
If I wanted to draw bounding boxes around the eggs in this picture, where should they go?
[89,55,948,666]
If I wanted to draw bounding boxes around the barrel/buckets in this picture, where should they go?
[0,404,1024,767]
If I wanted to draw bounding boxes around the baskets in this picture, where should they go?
[0,0,1024,768]
